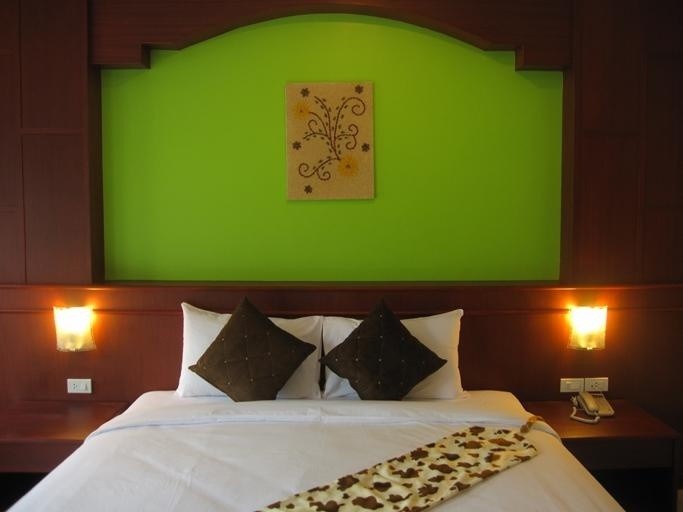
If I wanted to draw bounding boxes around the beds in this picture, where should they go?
[4,388,623,512]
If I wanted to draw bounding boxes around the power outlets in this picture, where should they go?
[583,377,609,392]
[65,377,92,394]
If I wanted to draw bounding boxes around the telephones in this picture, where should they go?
[576,390,615,417]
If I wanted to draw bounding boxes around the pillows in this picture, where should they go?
[175,292,464,401]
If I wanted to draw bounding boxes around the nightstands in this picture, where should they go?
[0,399,130,474]
[523,398,679,510]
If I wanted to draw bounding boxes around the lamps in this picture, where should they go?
[52,305,97,354]
[567,304,607,352]
